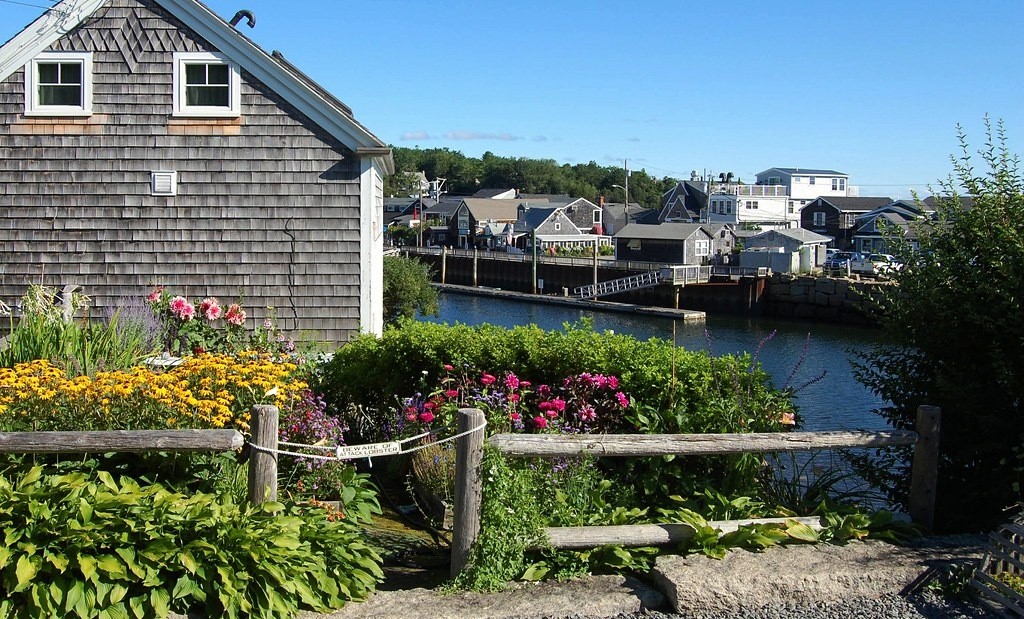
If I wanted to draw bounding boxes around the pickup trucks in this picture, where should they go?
[852,254,896,276]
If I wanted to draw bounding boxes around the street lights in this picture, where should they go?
[612,184,629,226]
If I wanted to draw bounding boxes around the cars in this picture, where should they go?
[822,251,861,275]
[825,248,843,262]
